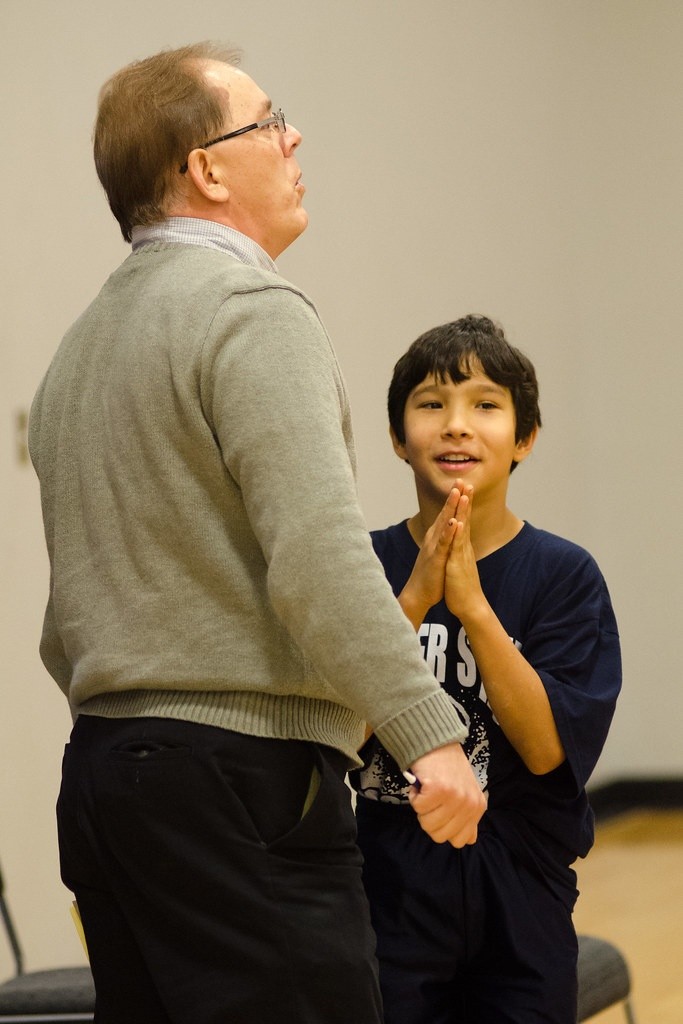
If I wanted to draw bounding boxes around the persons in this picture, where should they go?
[29,41,485,1024]
[350,315,623,1024]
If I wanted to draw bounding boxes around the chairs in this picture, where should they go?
[0,864,95,1024]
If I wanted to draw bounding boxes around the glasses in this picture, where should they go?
[179,109,285,173]
